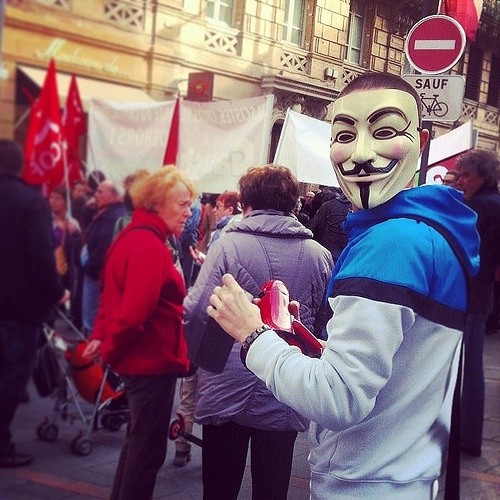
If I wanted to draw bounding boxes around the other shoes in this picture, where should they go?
[0,452,33,468]
[174,449,192,467]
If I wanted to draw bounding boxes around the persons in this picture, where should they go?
[458,148,500,458]
[441,170,461,188]
[181,164,335,500]
[167,191,242,463]
[206,71,482,500]
[296,184,352,258]
[83,164,202,500]
[47,171,151,405]
[0,138,64,464]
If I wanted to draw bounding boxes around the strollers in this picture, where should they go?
[38,300,185,461]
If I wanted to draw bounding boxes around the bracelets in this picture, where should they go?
[240,326,273,376]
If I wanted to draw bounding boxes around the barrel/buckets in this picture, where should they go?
[66,339,115,406]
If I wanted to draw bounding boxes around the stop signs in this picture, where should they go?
[405,14,467,76]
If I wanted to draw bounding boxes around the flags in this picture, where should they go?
[272,110,350,187]
[440,0,478,42]
[412,120,474,186]
[62,73,87,170]
[22,58,83,193]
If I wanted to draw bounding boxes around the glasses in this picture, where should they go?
[216,205,227,209]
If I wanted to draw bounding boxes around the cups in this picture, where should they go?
[258,279,297,339]
